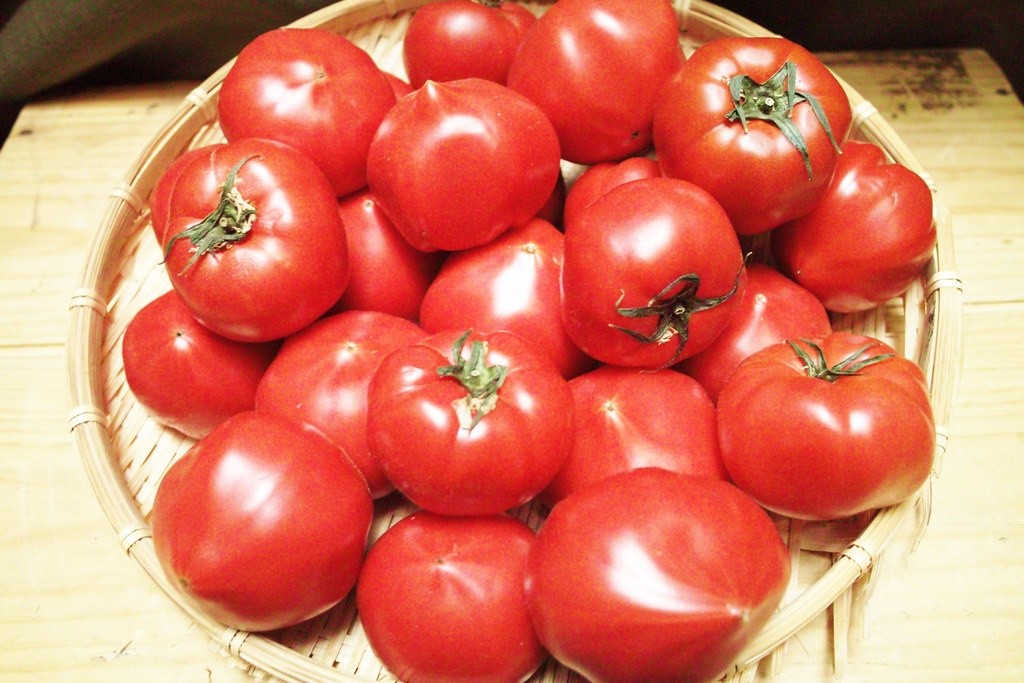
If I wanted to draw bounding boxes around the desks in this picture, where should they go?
[0,49,1024,683]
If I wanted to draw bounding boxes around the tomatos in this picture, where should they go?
[121,0,942,683]
